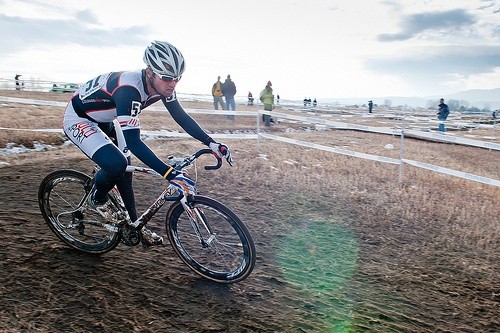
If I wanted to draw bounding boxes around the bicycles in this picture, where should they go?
[38,149,256,284]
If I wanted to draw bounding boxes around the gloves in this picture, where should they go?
[207,141,234,167]
[161,164,197,196]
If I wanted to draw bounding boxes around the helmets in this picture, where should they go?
[143,40,186,81]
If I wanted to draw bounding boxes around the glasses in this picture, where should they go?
[152,70,182,82]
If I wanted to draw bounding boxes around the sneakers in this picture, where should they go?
[130,222,164,244]
[87,189,128,224]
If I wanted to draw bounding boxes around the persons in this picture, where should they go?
[248,91,252,101]
[277,94,280,102]
[220,75,236,119]
[212,76,226,110]
[368,100,373,113]
[260,81,275,127]
[63,41,233,245]
[303,97,317,108]
[437,98,450,131]
